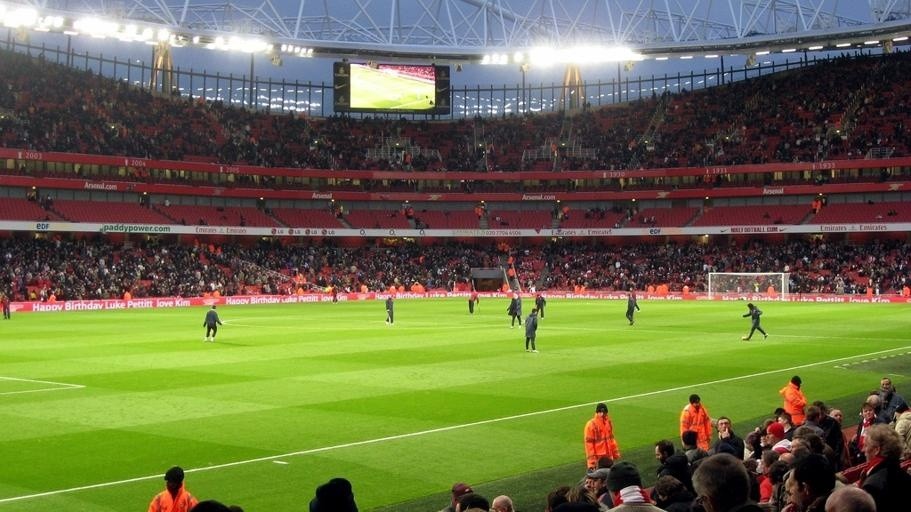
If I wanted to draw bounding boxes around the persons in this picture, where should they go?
[626,292,638,325]
[469,291,479,314]
[508,292,524,329]
[148,465,200,512]
[630,287,641,311]
[203,305,225,342]
[0,290,11,320]
[386,294,395,324]
[310,477,358,510]
[525,308,539,353]
[743,303,769,341]
[535,294,546,316]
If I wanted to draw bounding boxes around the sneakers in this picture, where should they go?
[205,337,214,342]
[763,333,769,339]
[526,349,538,352]
[743,337,750,341]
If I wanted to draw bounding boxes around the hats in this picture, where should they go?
[682,429,697,445]
[587,467,610,478]
[767,422,785,440]
[606,462,642,489]
[597,456,613,468]
[596,403,608,413]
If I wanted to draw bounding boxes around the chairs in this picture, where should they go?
[625,207,700,226]
[409,210,481,228]
[320,184,416,193]
[691,204,812,226]
[0,196,54,222]
[800,54,911,161]
[0,168,88,179]
[74,72,146,159]
[714,73,800,168]
[152,203,281,226]
[49,199,175,223]
[422,182,569,194]
[184,102,307,168]
[221,180,316,191]
[146,96,184,161]
[88,172,218,186]
[557,107,635,170]
[307,119,426,172]
[271,208,345,227]
[576,179,764,193]
[807,201,911,225]
[426,112,557,176]
[769,175,911,187]
[487,209,553,227]
[342,210,411,227]
[635,89,714,170]
[559,208,629,228]
[0,54,74,153]
